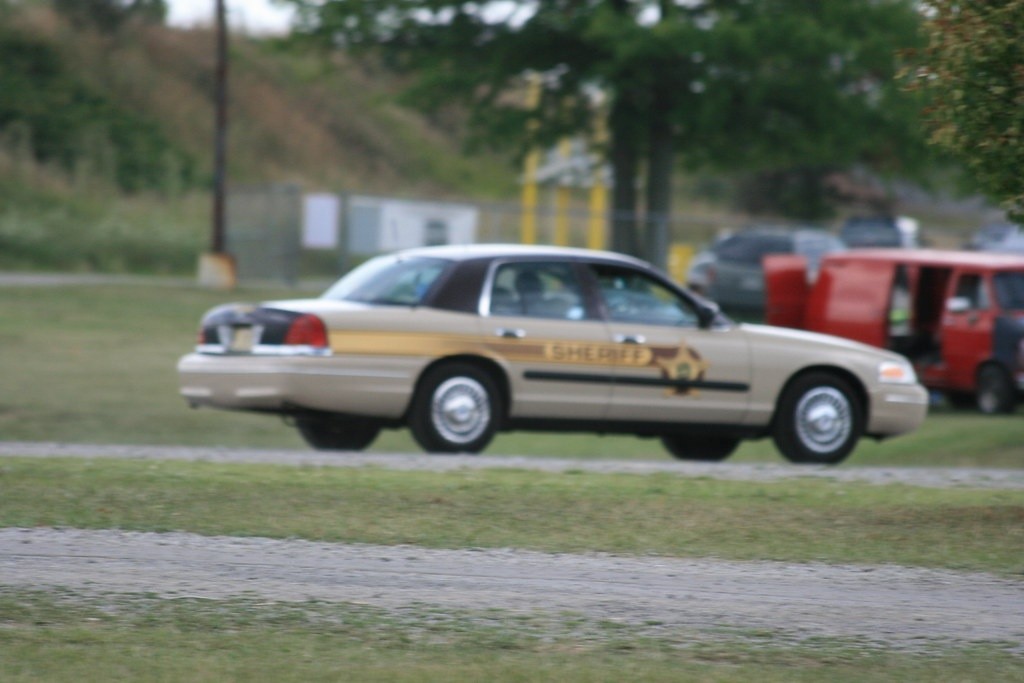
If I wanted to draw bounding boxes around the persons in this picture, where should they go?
[514,272,545,303]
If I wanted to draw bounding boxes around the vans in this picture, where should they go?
[842,216,923,247]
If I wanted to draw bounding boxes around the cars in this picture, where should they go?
[178,243,929,467]
[682,226,847,330]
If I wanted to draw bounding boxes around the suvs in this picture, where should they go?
[755,247,1024,419]
